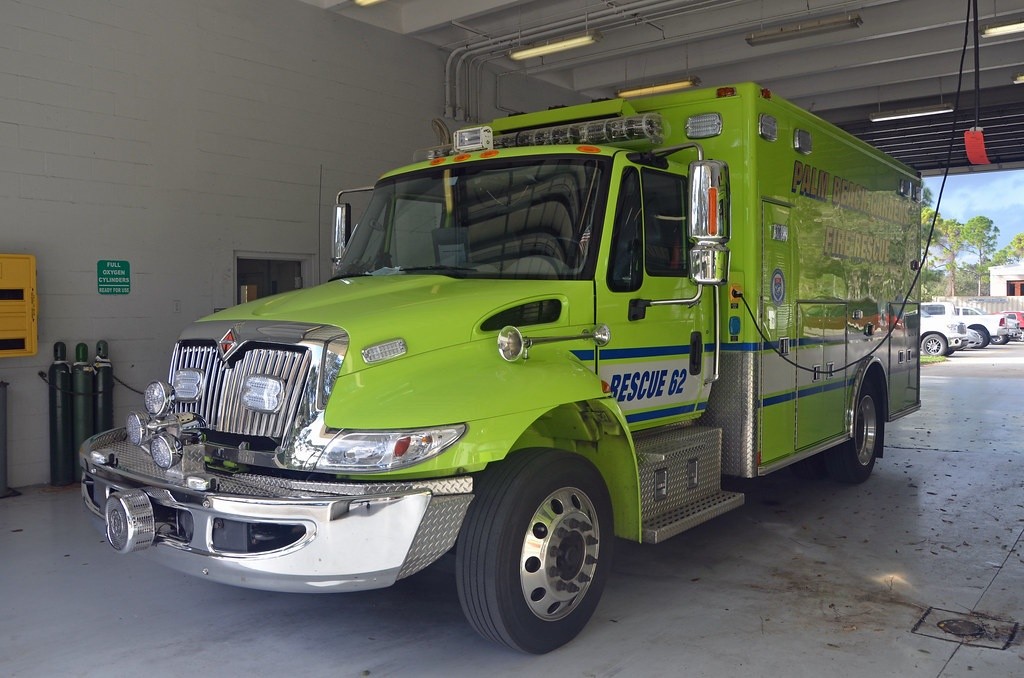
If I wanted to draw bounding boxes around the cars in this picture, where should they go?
[992,312,1024,329]
[956,307,1008,349]
[1007,318,1024,339]
[918,309,969,357]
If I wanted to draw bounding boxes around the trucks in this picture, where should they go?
[78,84,923,658]
